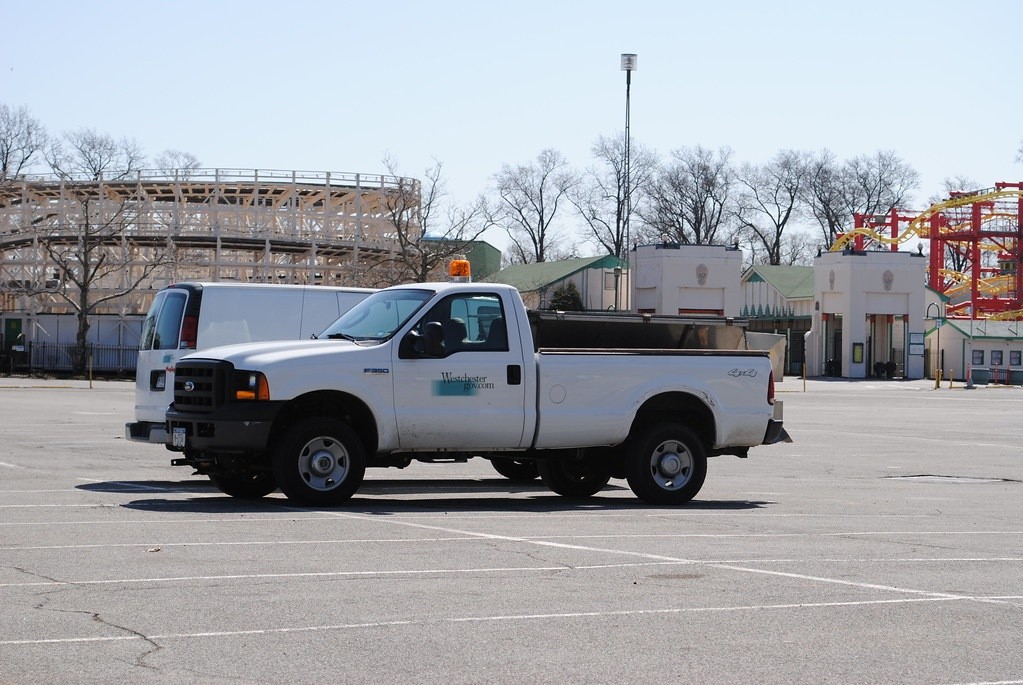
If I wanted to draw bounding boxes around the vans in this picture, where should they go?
[125,282,539,482]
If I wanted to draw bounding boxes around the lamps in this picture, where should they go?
[633,237,638,250]
[918,243,923,256]
[734,236,739,250]
[663,234,668,247]
[817,244,822,256]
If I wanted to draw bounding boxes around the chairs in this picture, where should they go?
[422,317,478,357]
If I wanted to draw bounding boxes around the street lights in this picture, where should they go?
[613,265,623,313]
[619,51,638,310]
[924,302,941,389]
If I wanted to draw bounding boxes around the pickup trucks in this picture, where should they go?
[160,260,795,510]
